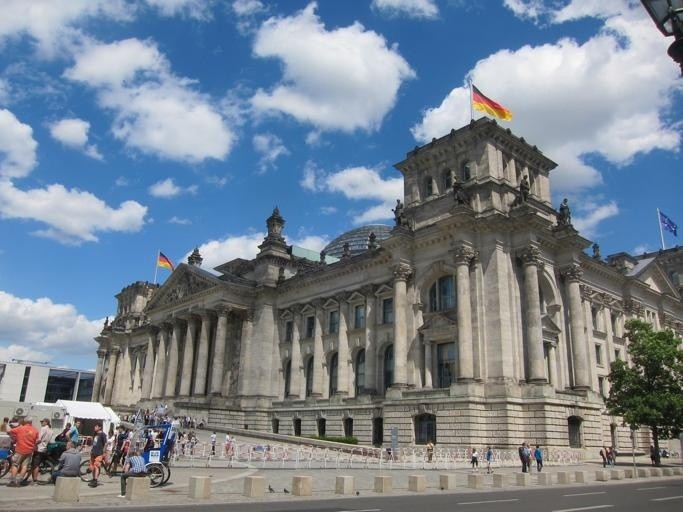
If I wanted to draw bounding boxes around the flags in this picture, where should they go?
[658,211,678,235]
[157,251,174,274]
[472,86,512,120]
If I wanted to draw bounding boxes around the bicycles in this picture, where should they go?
[668,449,678,459]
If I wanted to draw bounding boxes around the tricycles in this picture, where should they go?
[78,423,178,488]
[0,440,76,486]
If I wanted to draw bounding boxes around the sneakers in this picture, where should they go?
[117,494,126,498]
[88,481,97,487]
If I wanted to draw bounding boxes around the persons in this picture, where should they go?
[427,439,433,462]
[649,445,655,465]
[609,446,617,465]
[469,446,479,471]
[527,445,535,462]
[535,444,543,473]
[518,443,531,472]
[392,198,404,226]
[519,173,530,202]
[0,399,236,497]
[452,175,468,204]
[560,195,572,225]
[485,445,494,473]
